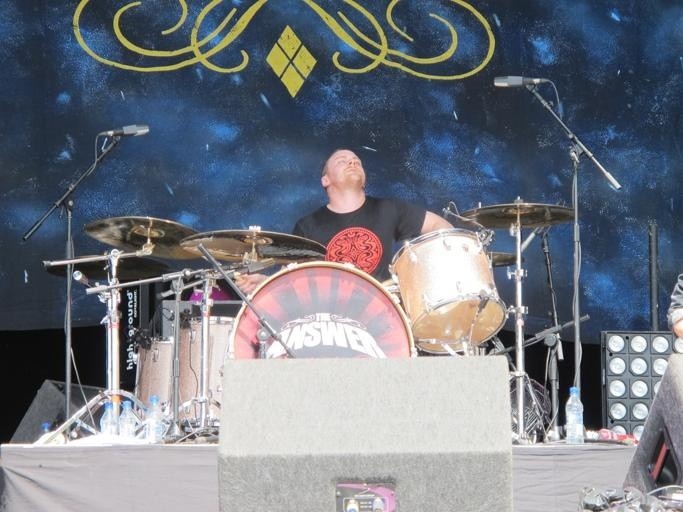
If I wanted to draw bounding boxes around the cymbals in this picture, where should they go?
[178,228,326,267]
[487,250,525,267]
[83,216,208,262]
[461,202,576,230]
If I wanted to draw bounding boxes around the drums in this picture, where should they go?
[175,314,233,434]
[134,336,177,425]
[389,228,508,355]
[232,259,416,358]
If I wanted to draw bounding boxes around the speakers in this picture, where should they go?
[8,379,137,444]
[217,356,513,512]
[620,353,682,497]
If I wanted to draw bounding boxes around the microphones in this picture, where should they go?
[99,124,150,137]
[494,75,541,88]
[226,261,264,278]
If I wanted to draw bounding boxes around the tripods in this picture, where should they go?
[506,168,630,447]
[37,262,222,444]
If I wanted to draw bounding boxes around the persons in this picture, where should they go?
[668,270,683,339]
[292,150,456,288]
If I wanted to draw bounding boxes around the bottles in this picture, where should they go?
[145,394,163,443]
[118,400,136,436]
[564,387,584,443]
[36,422,51,440]
[99,401,117,438]
